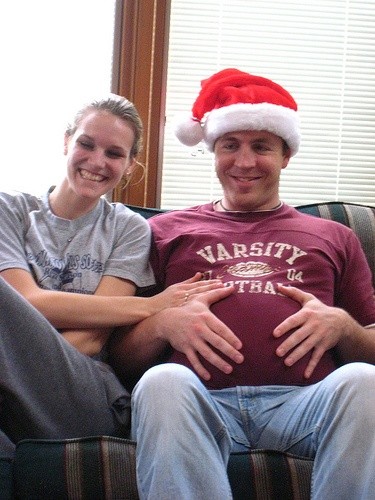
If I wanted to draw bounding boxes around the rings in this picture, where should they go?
[183,294,188,303]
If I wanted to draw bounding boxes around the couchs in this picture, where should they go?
[14,203,375,500]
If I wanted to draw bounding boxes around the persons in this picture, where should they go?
[118,68,375,499]
[0,93,225,457]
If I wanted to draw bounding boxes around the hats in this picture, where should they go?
[173,65,302,156]
